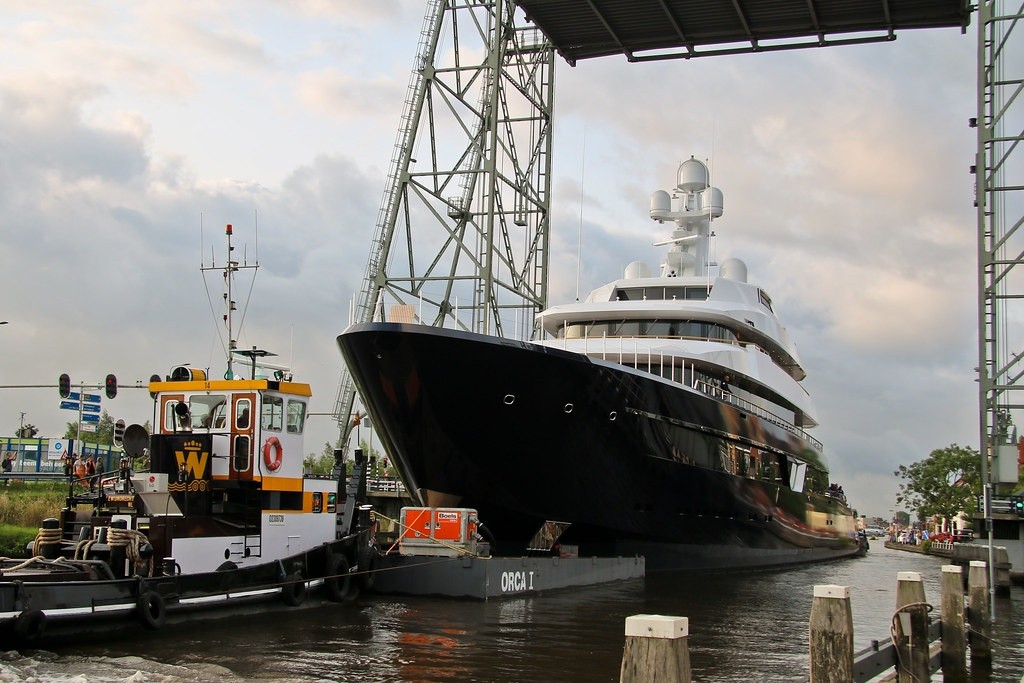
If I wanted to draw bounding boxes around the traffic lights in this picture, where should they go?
[113,417,126,448]
[60,373,71,398]
[149,374,162,401]
[106,373,117,399]
[1016,495,1023,517]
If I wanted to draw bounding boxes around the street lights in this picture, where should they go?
[169,363,191,376]
[205,367,210,380]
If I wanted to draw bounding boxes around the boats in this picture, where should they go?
[0,208,352,648]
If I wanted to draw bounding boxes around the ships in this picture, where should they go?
[334,155,870,581]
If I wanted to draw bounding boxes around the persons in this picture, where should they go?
[61,450,104,491]
[238,409,249,427]
[4,452,17,484]
[831,483,845,494]
[201,414,212,428]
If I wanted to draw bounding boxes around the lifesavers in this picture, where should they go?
[263,436,284,471]
